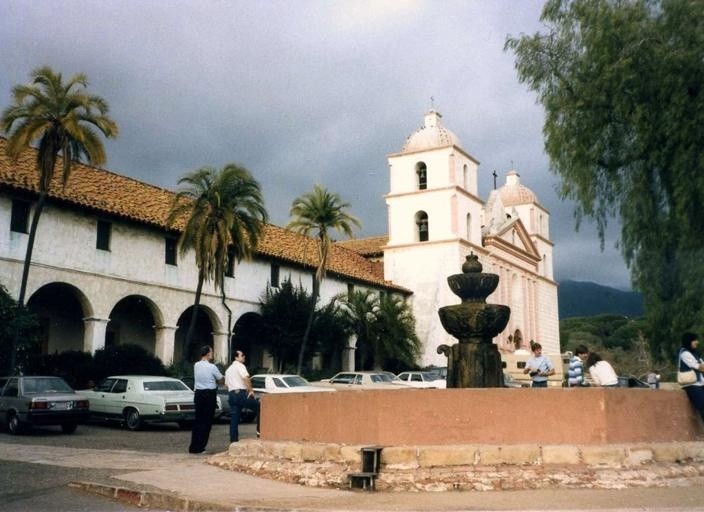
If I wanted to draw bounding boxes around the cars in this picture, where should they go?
[310,369,406,388]
[429,367,447,378]
[248,373,337,400]
[73,374,223,431]
[395,371,446,389]
[180,375,257,424]
[0,376,89,436]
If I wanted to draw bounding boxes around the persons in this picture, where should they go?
[190,345,260,455]
[647,369,660,389]
[524,343,619,387]
[677,333,704,423]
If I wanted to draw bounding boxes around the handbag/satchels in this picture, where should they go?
[677,370,697,386]
